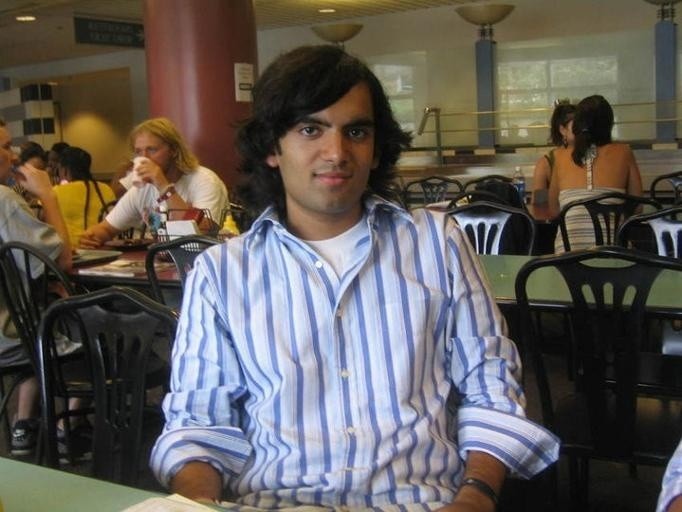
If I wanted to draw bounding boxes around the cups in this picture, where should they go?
[132,156,151,188]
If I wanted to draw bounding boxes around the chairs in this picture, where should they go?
[0,173,681,512]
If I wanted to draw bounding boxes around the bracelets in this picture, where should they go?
[158,183,179,205]
[457,477,502,508]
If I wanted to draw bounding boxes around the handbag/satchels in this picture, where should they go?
[471,181,533,255]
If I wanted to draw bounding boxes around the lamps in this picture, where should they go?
[309,20,364,54]
[453,4,516,43]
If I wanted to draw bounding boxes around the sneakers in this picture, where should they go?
[56,418,93,465]
[12,414,36,455]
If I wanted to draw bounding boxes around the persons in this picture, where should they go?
[147,42,562,512]
[654,436,682,512]
[1,118,121,467]
[38,143,117,248]
[10,136,51,222]
[75,114,240,250]
[527,102,580,222]
[44,140,71,185]
[545,92,644,259]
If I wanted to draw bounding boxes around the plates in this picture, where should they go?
[104,238,153,251]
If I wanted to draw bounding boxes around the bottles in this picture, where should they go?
[222,214,240,236]
[512,166,526,205]
